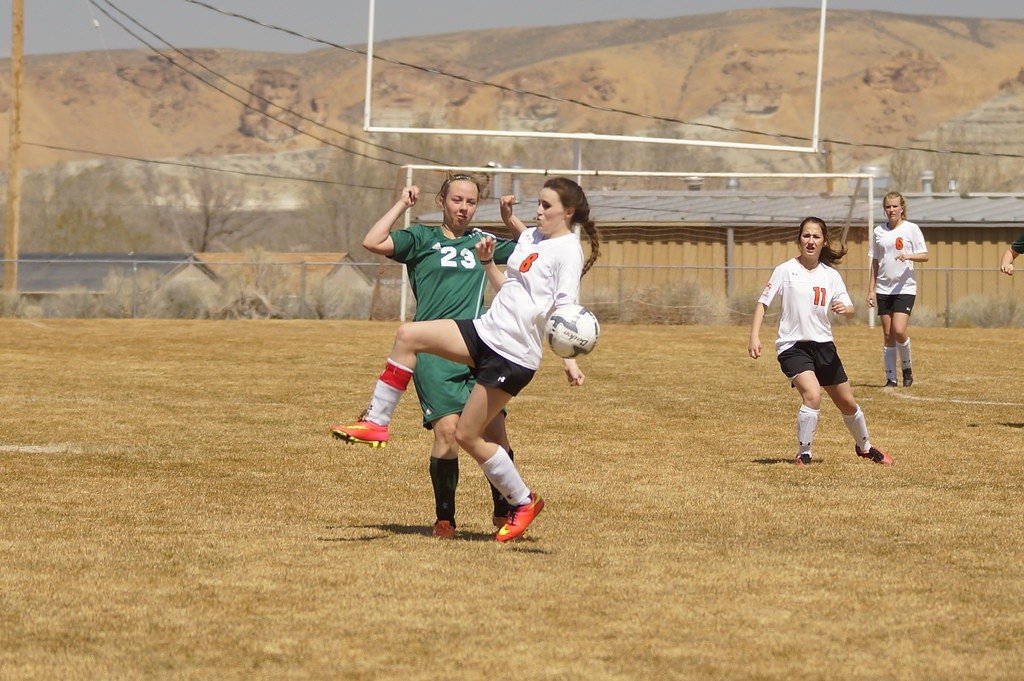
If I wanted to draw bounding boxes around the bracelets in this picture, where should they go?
[480,257,493,265]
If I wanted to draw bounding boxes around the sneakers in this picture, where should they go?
[795,453,811,465]
[432,516,456,540]
[855,442,895,467]
[495,491,546,542]
[492,516,506,528]
[883,378,897,387]
[326,419,389,449]
[901,366,913,387]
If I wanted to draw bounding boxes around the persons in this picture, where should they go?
[330,172,599,542]
[748,216,895,466]
[865,192,928,388]
[1000,230,1024,275]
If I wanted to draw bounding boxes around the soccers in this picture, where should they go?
[545,303,602,360]
